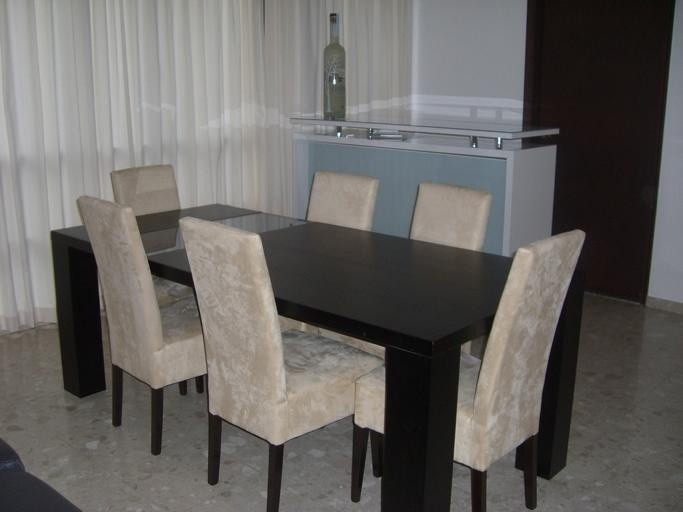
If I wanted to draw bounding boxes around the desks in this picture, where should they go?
[45,199,585,510]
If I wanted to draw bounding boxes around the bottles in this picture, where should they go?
[322,10,346,120]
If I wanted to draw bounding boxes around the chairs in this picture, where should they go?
[106,162,204,395]
[343,178,493,355]
[72,197,204,456]
[281,164,380,336]
[351,225,586,510]
[179,215,385,510]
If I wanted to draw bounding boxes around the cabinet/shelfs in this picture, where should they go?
[289,111,560,258]
